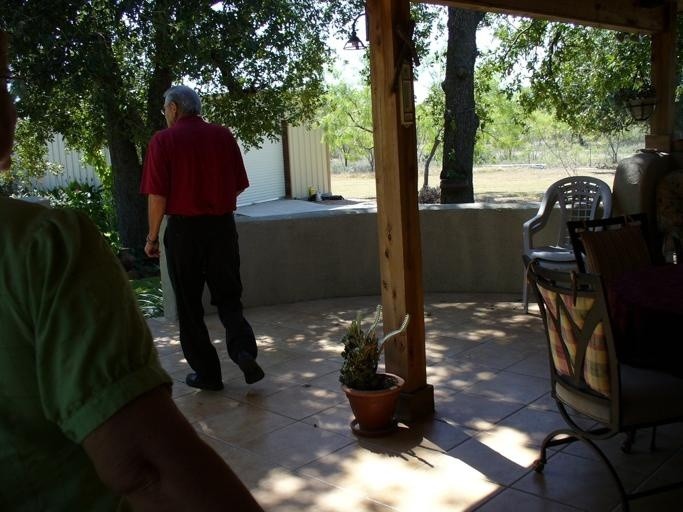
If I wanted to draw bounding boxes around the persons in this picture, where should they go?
[0,73,267,512]
[654,137,683,266]
[138,83,266,392]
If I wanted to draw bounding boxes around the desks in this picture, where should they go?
[606,264,682,377]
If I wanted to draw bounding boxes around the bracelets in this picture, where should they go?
[145,235,160,245]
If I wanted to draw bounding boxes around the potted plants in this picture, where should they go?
[611,62,661,124]
[335,301,414,440]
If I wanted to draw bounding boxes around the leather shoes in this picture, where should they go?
[237,352,264,384]
[186,373,223,391]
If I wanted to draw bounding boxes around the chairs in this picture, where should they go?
[568,210,667,275]
[641,153,681,264]
[520,254,681,510]
[524,176,614,314]
